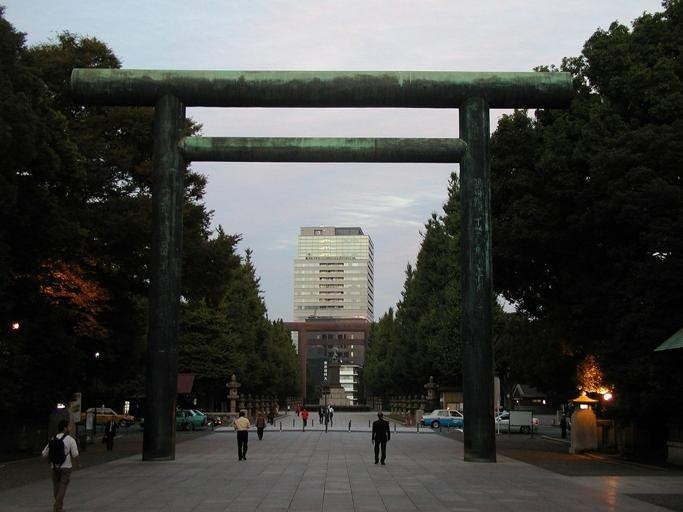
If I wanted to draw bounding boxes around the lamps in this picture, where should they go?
[567,391,599,410]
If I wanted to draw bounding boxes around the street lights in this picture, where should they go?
[93,349,100,437]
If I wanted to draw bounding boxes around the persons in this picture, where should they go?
[233,411,251,461]
[41,419,81,512]
[295,403,334,426]
[254,411,265,440]
[372,412,390,465]
[559,413,568,439]
[103,415,117,455]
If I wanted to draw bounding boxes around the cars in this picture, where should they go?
[78,403,135,429]
[417,407,463,431]
[494,412,540,435]
[137,409,225,433]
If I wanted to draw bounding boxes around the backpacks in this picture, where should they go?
[48,432,71,465]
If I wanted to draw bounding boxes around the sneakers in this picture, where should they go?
[375,459,385,465]
[239,456,246,460]
